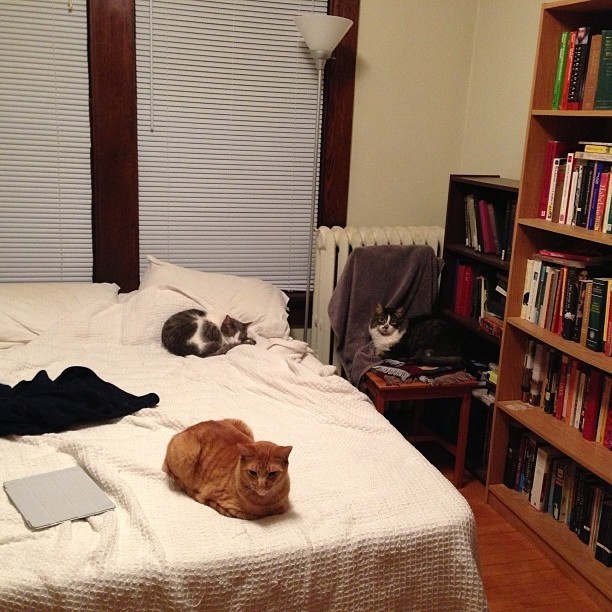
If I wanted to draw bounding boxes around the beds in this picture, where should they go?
[0,279,494,611]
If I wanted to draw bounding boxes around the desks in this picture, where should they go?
[364,370,477,485]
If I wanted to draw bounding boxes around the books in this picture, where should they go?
[463,193,518,263]
[536,137,612,236]
[550,26,612,110]
[449,258,508,341]
[520,241,612,359]
[502,423,612,569]
[518,336,612,452]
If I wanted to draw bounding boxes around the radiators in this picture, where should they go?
[309,226,446,379]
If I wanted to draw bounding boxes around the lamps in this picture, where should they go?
[288,10,356,341]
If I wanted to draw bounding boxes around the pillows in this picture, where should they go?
[137,255,289,335]
[0,282,118,341]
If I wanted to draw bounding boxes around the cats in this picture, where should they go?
[368,303,477,376]
[162,309,256,358]
[161,417,292,520]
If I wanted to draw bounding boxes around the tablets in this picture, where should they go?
[2,465,117,531]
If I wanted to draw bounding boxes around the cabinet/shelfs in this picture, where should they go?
[440,174,524,484]
[487,0,612,602]
